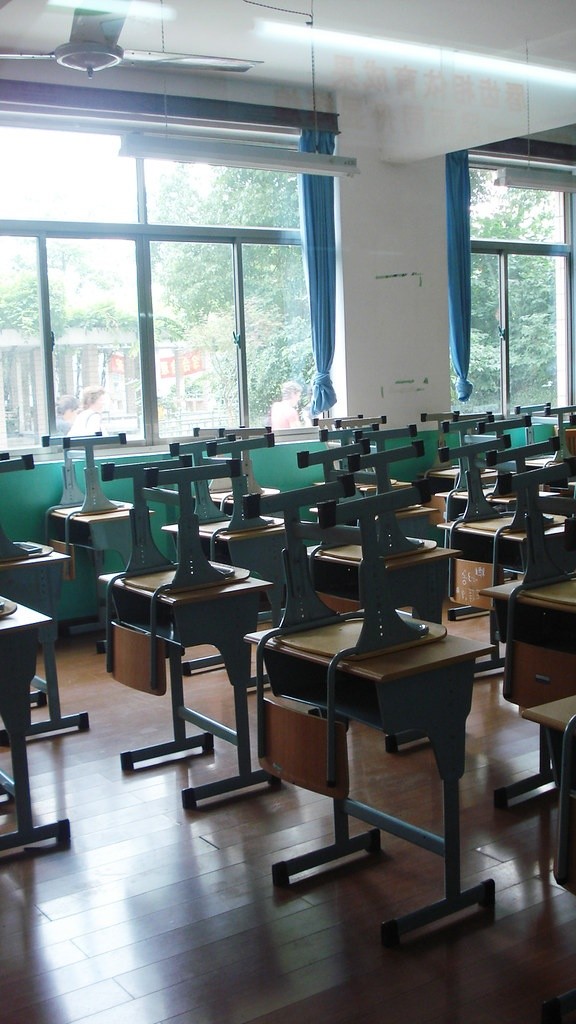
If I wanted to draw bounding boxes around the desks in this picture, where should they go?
[0,458,575,1024]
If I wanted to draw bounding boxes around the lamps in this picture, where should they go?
[116,0,362,179]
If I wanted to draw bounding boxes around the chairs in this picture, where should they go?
[0,400,576,897]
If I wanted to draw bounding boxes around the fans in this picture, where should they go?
[0,0,267,81]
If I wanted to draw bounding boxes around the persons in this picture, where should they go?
[271,381,302,429]
[56,386,110,436]
